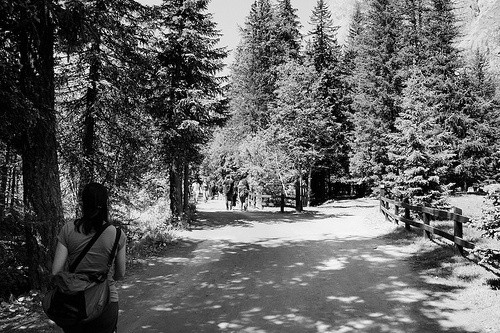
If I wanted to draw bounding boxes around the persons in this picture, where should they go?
[51,182,127,333]
[191,172,251,212]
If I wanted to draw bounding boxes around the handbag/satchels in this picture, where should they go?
[42,271,108,326]
